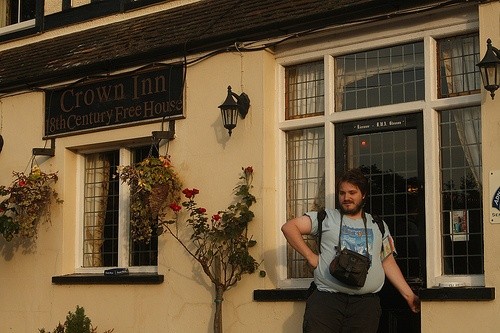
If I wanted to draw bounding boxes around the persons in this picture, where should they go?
[281,169,421,333]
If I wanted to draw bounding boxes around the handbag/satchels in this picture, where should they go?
[329,212,371,286]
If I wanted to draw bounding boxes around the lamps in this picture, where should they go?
[474,38,500,100]
[217,85,251,136]
